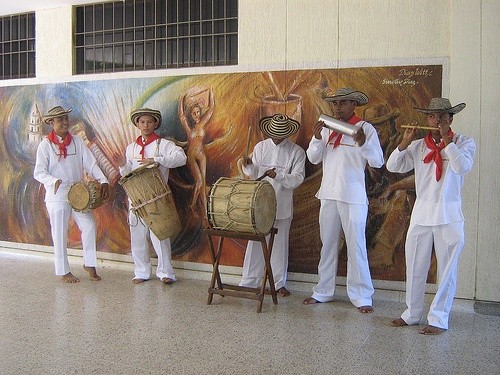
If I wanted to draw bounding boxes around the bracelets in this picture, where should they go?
[442,135,450,140]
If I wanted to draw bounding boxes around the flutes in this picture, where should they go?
[400,124,440,130]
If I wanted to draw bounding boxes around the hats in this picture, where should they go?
[130,108,161,130]
[43,106,73,125]
[322,88,369,106]
[259,114,300,140]
[413,98,466,115]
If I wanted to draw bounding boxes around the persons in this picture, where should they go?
[33,105,110,284]
[238,114,306,297]
[386,98,476,336]
[303,88,385,313]
[119,108,188,284]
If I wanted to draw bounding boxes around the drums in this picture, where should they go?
[118,163,181,241]
[68,181,116,213]
[206,176,277,236]
